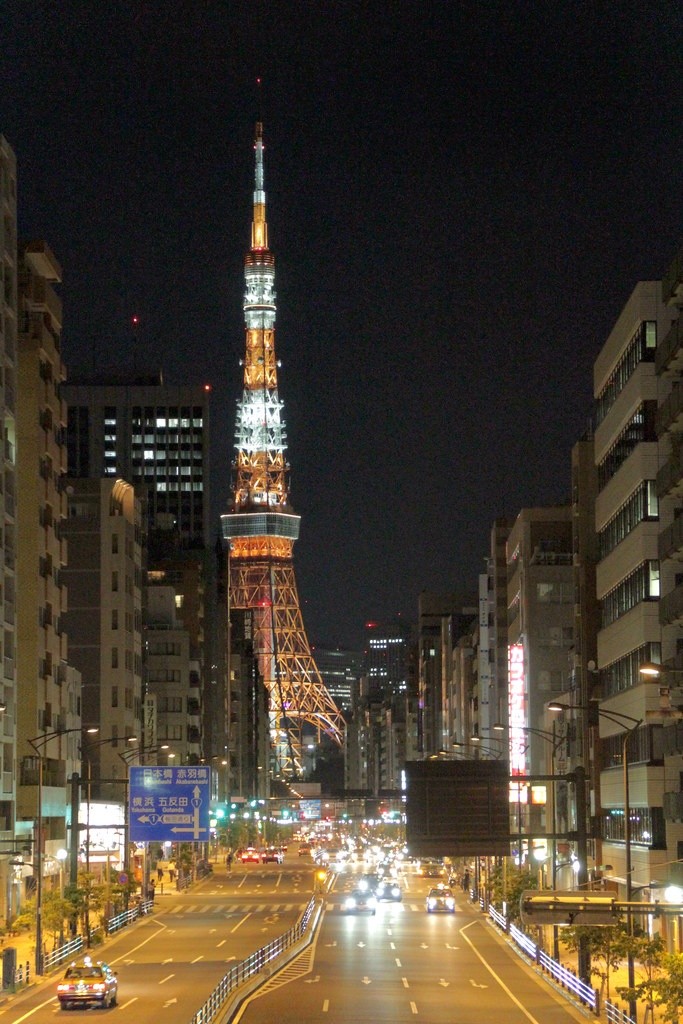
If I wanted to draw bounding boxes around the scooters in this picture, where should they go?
[448,873,457,888]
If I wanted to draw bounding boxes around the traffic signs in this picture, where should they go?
[128,765,210,843]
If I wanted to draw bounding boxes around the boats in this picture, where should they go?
[426,888,456,913]
[240,827,444,916]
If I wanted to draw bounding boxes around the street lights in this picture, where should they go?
[548,701,644,1024]
[470,734,529,871]
[28,727,99,974]
[494,722,568,983]
[77,734,141,949]
[117,744,170,928]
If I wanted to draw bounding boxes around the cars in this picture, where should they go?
[56,961,120,1010]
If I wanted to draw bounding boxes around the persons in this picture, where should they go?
[227,854,232,867]
[149,879,155,899]
[168,860,175,882]
[226,863,231,878]
[157,860,162,881]
[464,868,469,892]
